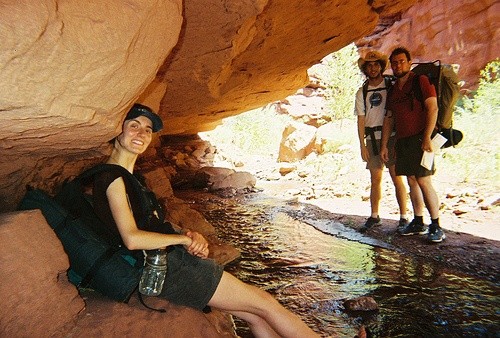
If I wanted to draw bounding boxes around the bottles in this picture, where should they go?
[138,246,167,297]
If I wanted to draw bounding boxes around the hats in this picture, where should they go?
[357,51,387,72]
[107,102,163,143]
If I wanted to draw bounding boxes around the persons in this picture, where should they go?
[91,104,324,337]
[353,48,411,234]
[380,49,449,243]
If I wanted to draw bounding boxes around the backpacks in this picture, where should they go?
[410,60,459,128]
[19,188,144,301]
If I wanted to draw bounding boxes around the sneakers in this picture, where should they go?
[426,224,446,243]
[401,221,428,236]
[397,218,408,233]
[363,215,382,232]
